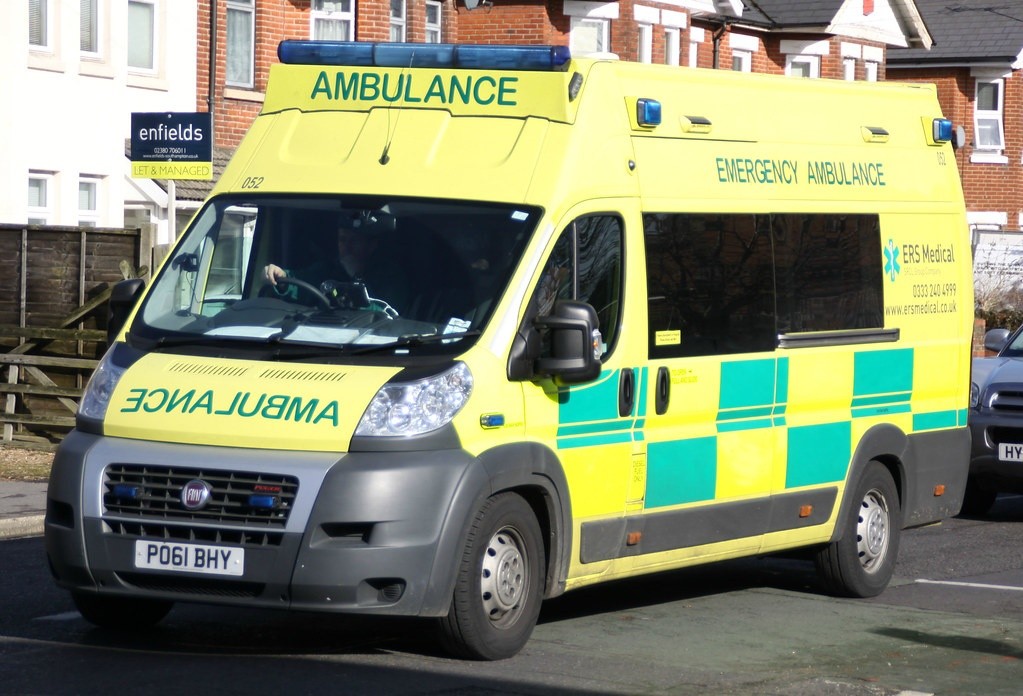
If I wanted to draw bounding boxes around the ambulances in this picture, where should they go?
[43,36,977,665]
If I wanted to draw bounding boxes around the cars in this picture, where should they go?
[968,317,1023,507]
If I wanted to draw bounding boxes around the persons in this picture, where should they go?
[262,206,386,310]
